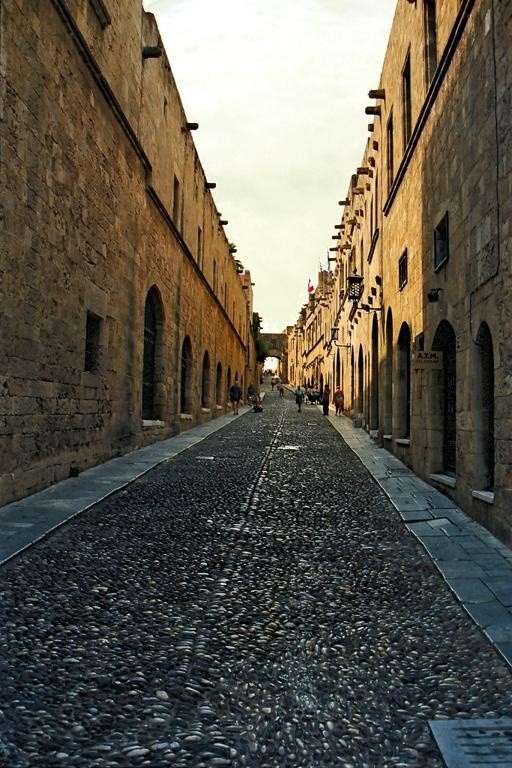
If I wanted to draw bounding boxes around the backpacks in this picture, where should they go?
[335,395,344,406]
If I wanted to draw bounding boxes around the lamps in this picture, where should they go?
[346,272,382,311]
[331,327,351,347]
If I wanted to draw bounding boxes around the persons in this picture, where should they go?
[332,386,344,416]
[320,385,331,415]
[230,381,242,416]
[260,369,284,397]
[294,386,305,412]
[304,386,321,405]
[247,383,255,395]
